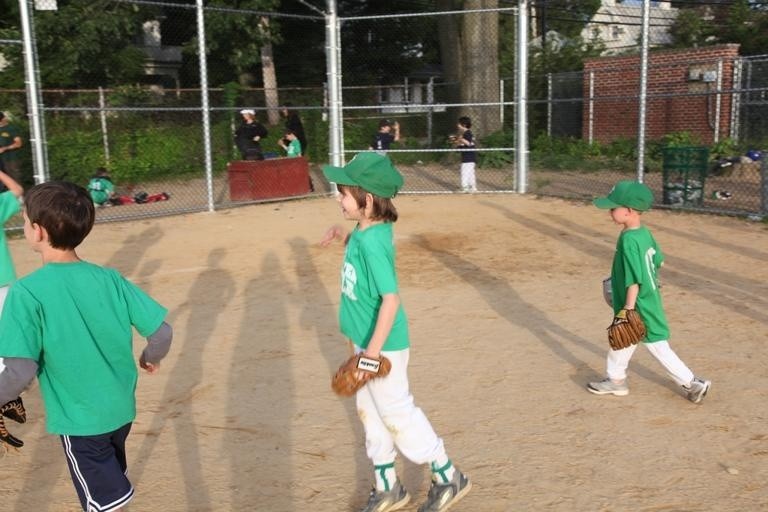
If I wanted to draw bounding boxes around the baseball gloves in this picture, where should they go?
[333,353,390,394]
[606,310,645,351]
[0,394,28,448]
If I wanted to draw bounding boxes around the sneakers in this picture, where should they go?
[361,476,411,512]
[682,378,711,406]
[587,378,629,397]
[416,471,472,512]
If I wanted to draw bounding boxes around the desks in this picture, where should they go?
[224,156,313,202]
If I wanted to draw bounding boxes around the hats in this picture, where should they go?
[593,181,655,213]
[240,110,256,116]
[321,153,404,199]
[379,120,394,128]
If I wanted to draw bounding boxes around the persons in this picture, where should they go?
[234,109,269,160]
[0,169,24,377]
[280,103,315,192]
[321,150,471,512]
[0,180,172,511]
[447,117,477,192]
[0,111,25,207]
[86,168,116,209]
[277,129,302,158]
[369,118,400,150]
[584,180,711,404]
